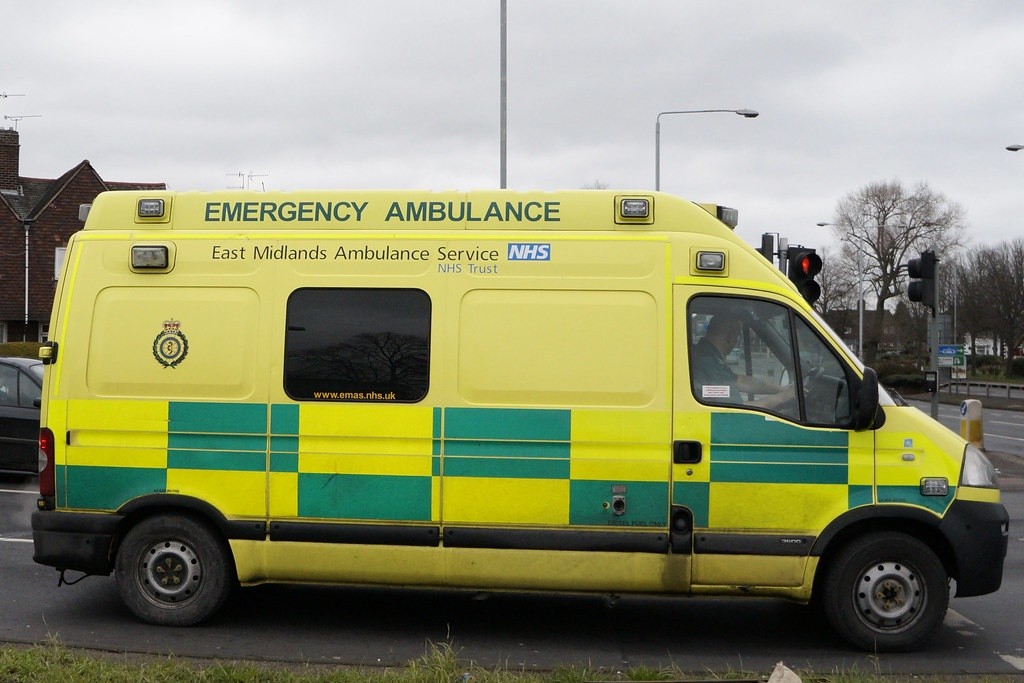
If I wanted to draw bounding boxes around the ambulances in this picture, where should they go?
[30,188,1010,652]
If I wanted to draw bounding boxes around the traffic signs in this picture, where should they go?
[938,356,966,367]
[938,344,964,356]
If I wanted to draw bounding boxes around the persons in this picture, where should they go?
[692,314,809,415]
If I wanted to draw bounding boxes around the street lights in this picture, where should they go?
[816,221,907,366]
[787,247,823,306]
[655,107,759,193]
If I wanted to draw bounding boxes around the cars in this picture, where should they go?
[0,356,46,476]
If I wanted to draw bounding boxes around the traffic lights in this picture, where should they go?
[908,251,935,304]
